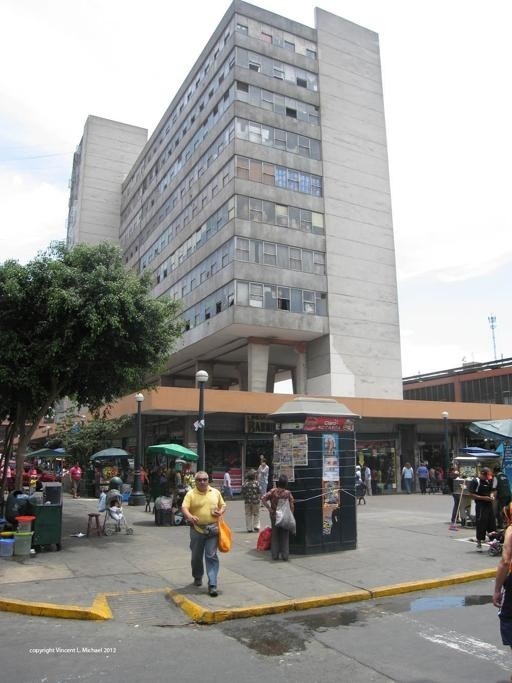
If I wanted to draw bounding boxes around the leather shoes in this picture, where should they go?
[194,577,203,586]
[208,586,218,596]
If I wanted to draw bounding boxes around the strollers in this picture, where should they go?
[102,488,135,536]
[486,500,512,556]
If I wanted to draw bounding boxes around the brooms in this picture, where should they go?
[449,479,465,531]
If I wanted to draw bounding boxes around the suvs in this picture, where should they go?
[0,459,58,490]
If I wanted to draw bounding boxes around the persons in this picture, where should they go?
[449,464,511,550]
[491,501,512,651]
[2,458,39,495]
[261,475,295,562]
[354,461,443,496]
[111,502,124,521]
[141,464,148,486]
[70,458,82,498]
[241,468,262,532]
[182,469,227,597]
[257,458,269,507]
[224,468,233,500]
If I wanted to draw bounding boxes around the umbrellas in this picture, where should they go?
[146,442,201,473]
[91,446,133,467]
[54,446,75,460]
[466,415,512,470]
[457,443,500,463]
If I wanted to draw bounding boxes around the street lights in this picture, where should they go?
[487,314,498,362]
[44,425,51,439]
[441,409,452,494]
[80,415,86,426]
[127,390,148,506]
[194,367,210,473]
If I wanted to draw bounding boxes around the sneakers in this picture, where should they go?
[476,545,482,553]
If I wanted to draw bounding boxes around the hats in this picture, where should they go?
[480,467,492,475]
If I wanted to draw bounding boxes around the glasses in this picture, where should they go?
[195,477,208,483]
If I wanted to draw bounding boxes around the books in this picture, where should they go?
[272,433,308,481]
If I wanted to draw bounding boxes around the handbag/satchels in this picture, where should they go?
[200,524,217,537]
[218,517,232,551]
[257,527,271,550]
[275,498,298,535]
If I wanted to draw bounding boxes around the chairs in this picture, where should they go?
[356,482,367,505]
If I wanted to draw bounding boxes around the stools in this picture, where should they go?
[87,512,102,537]
